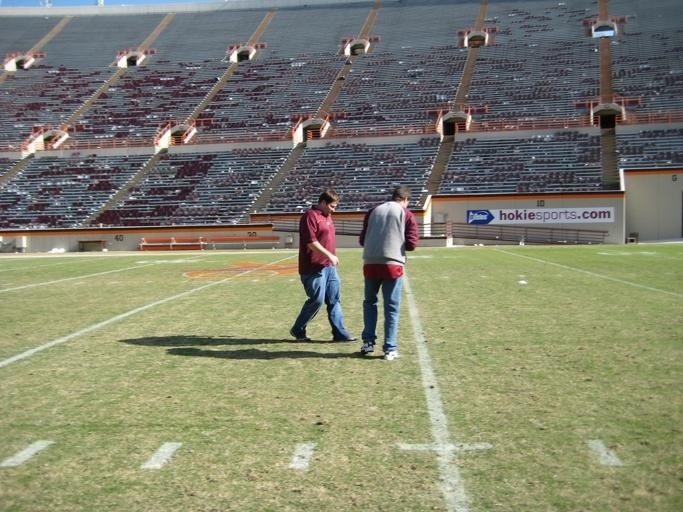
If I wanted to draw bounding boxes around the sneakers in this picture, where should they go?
[361,341,374,354]
[347,337,358,342]
[384,350,400,361]
[290,329,310,341]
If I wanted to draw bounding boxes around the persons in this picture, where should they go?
[290,190,358,342]
[359,185,419,360]
